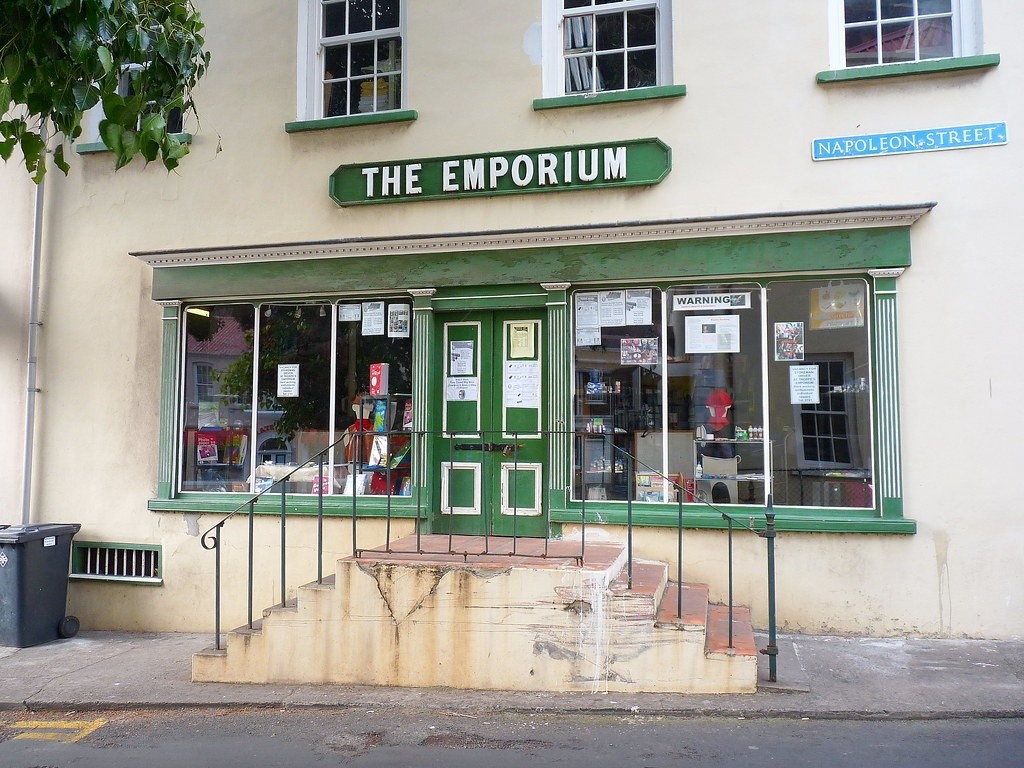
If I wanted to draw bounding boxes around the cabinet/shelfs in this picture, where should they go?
[357,394,412,496]
[693,437,775,504]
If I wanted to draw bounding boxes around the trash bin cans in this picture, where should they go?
[0,523,82,649]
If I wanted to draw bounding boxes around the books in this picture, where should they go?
[369,363,389,396]
[198,433,248,465]
[342,474,368,496]
[312,475,328,495]
[369,399,412,467]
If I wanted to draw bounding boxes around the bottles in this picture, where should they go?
[695,461,703,478]
[748,425,763,442]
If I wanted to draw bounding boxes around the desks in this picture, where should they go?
[245,463,348,494]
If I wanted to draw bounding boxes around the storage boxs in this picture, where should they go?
[232,481,250,492]
[369,363,390,395]
